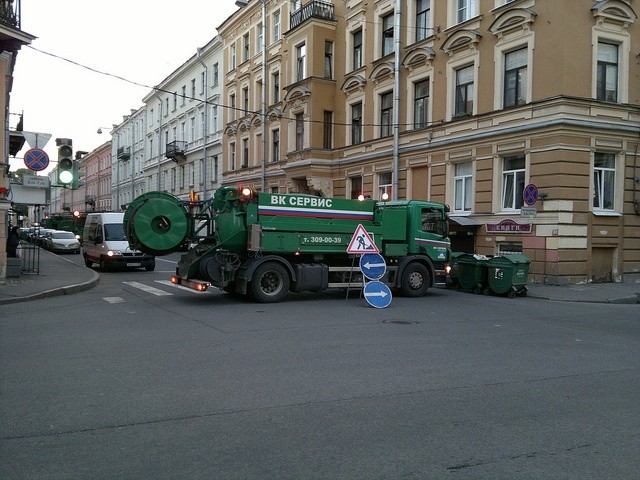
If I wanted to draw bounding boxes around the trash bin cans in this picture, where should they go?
[445,252,465,291]
[455,253,488,295]
[484,255,531,298]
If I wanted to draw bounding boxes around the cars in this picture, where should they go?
[42,231,80,253]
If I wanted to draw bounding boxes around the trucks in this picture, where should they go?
[123,186,451,301]
[43,212,76,233]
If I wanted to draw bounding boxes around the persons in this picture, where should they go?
[6,226,20,258]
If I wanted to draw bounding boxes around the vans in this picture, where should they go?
[83,213,155,271]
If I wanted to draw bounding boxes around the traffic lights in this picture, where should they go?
[58,145,73,185]
